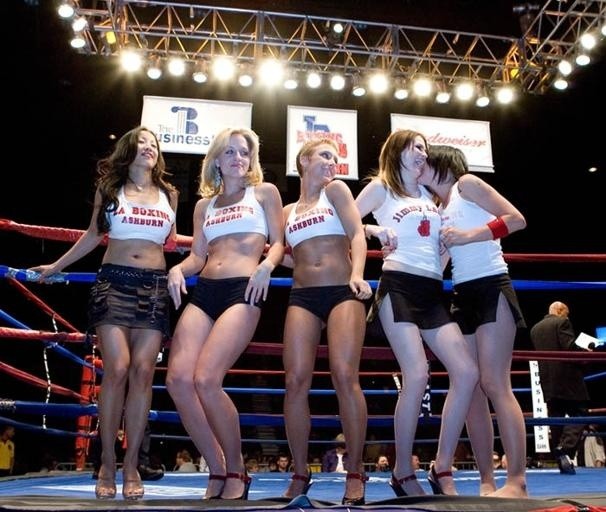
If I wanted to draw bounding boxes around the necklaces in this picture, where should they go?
[131,180,151,192]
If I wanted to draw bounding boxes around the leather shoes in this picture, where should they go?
[90,467,99,480]
[135,465,163,480]
[555,445,575,476]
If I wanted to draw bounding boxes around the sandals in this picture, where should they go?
[337,472,368,507]
[203,467,254,499]
[122,469,144,499]
[276,470,315,501]
[92,470,117,500]
[429,464,454,501]
[388,470,426,506]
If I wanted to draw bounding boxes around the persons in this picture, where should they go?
[574,428,606,468]
[276,456,289,472]
[175,450,197,473]
[412,455,424,471]
[323,433,348,473]
[0,427,15,476]
[92,401,163,481]
[375,455,390,472]
[27,126,178,500]
[268,459,277,471]
[354,129,479,498]
[530,301,591,474]
[280,137,372,508]
[492,454,503,470]
[166,127,282,502]
[416,145,528,500]
[246,458,259,473]
